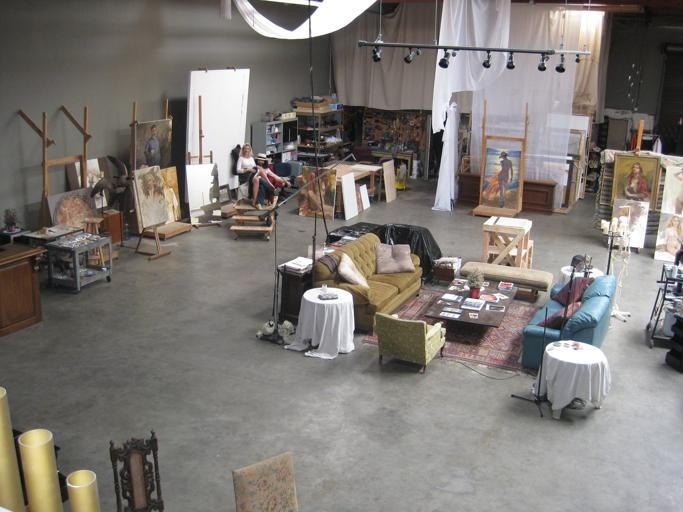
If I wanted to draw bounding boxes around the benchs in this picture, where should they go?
[460,263,554,303]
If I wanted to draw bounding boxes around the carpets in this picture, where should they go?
[364,287,546,378]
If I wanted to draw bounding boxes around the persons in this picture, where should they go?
[496,151,514,208]
[624,161,649,201]
[664,215,683,256]
[160,127,173,167]
[620,201,642,233]
[138,169,169,227]
[143,124,161,166]
[235,143,275,207]
[252,152,292,210]
[161,170,179,225]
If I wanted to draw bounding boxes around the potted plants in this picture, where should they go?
[4,206,17,231]
[466,266,484,299]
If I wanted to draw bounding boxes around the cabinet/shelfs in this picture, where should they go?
[646,265,683,349]
[252,119,297,163]
[294,111,344,163]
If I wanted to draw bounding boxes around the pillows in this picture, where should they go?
[553,276,597,306]
[538,302,583,328]
[374,244,415,274]
[338,253,371,290]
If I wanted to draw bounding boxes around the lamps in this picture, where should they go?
[358,0,591,73]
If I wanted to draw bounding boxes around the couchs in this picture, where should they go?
[314,233,422,337]
[522,276,617,370]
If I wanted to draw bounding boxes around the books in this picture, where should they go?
[441,278,514,311]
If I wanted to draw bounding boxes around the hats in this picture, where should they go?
[499,152,509,158]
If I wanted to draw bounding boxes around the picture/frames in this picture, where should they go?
[611,152,662,212]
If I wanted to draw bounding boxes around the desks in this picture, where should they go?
[279,271,310,328]
[533,340,613,421]
[284,287,356,359]
[5,230,33,246]
[372,149,413,181]
[297,161,382,219]
[25,225,88,248]
[45,231,113,294]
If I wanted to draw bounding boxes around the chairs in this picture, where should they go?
[376,313,447,374]
